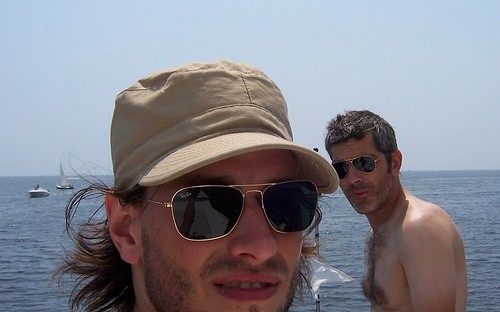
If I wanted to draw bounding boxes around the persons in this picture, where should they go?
[324,109,468,312]
[50,60,341,312]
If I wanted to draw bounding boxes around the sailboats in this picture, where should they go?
[56,161,74,189]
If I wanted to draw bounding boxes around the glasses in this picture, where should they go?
[331,152,385,179]
[137,176,320,243]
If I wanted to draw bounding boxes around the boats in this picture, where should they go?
[28,185,50,196]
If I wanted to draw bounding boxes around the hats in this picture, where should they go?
[109,60,340,194]
